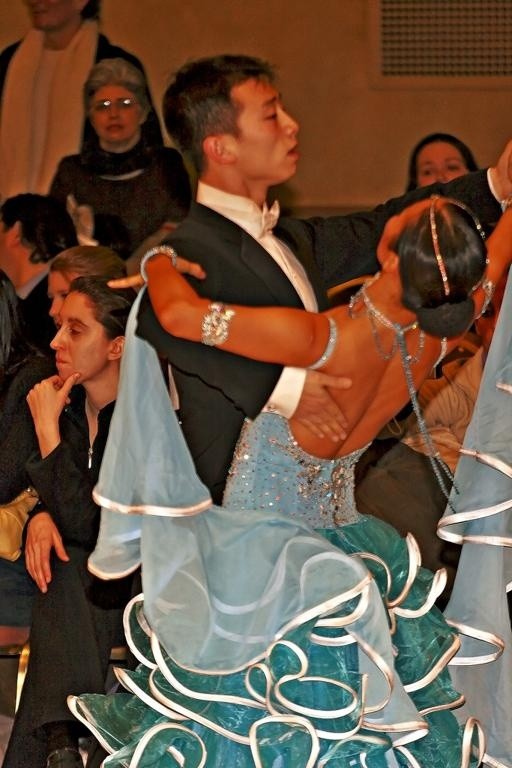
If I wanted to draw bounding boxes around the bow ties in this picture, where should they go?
[256,200,282,238]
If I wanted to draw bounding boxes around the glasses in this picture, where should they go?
[90,97,149,114]
